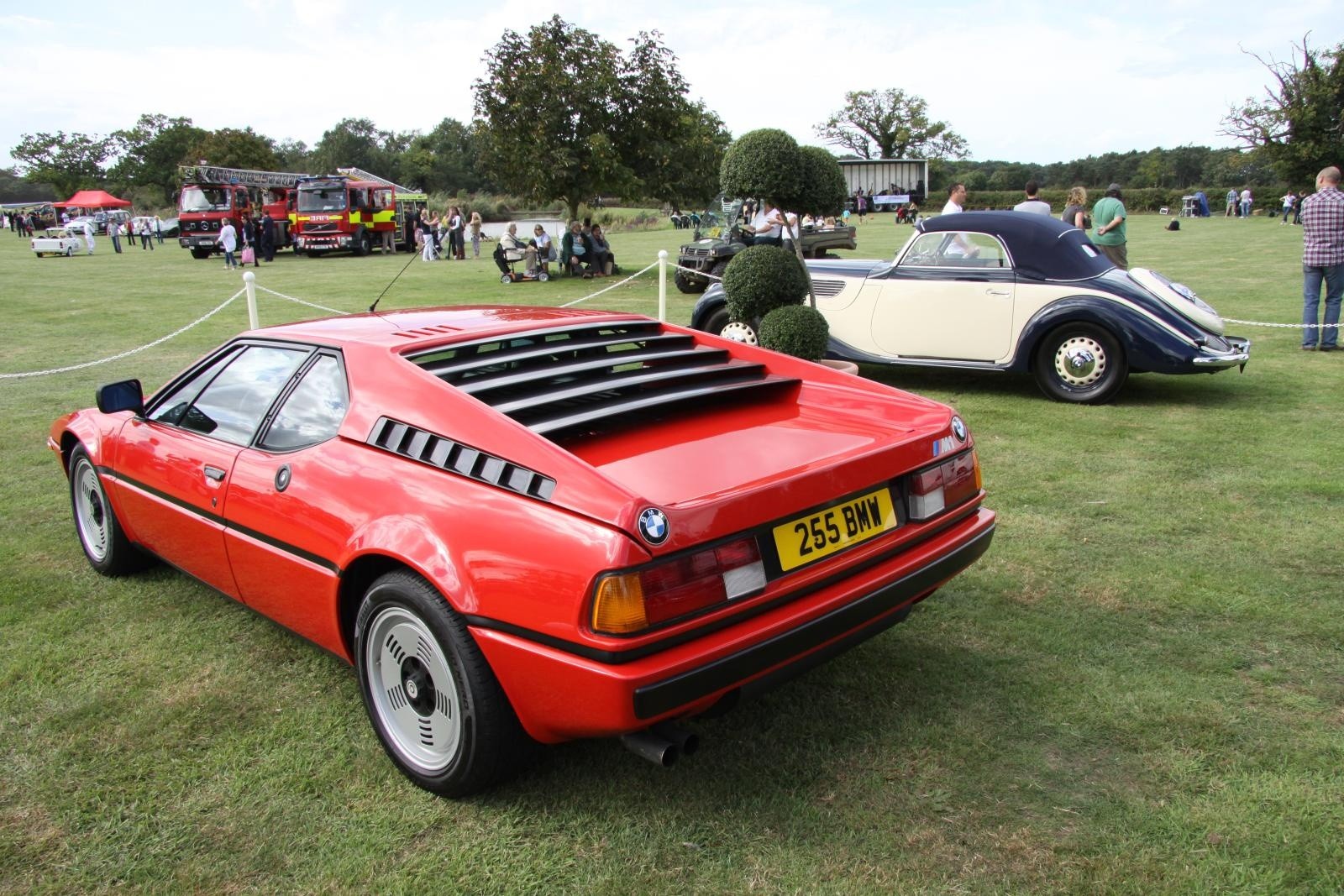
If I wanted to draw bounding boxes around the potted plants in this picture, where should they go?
[721,129,859,376]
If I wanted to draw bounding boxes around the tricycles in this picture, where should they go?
[493,239,550,285]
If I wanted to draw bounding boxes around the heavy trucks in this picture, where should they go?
[837,159,929,213]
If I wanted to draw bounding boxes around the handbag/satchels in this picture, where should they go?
[515,241,527,248]
[241,244,255,263]
[604,261,613,276]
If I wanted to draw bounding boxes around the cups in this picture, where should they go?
[577,238,581,244]
[600,234,605,240]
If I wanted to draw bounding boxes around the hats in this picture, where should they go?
[1298,190,1306,197]
[155,216,161,220]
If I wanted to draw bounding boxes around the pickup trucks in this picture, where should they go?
[674,190,858,294]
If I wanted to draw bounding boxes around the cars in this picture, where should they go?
[685,211,1250,405]
[26,204,179,238]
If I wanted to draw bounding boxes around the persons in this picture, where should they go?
[1299,166,1344,351]
[1059,186,1087,233]
[895,201,918,225]
[746,199,798,251]
[560,216,615,279]
[470,211,482,259]
[381,229,397,255]
[404,203,443,261]
[533,224,557,270]
[1091,183,1128,270]
[940,180,975,259]
[1224,188,1240,218]
[441,206,465,260]
[671,209,719,229]
[1280,189,1308,225]
[499,221,538,278]
[84,218,154,255]
[214,210,277,271]
[8,207,87,237]
[1014,179,1050,217]
[152,216,164,243]
[801,208,851,229]
[856,189,867,224]
[1239,185,1251,218]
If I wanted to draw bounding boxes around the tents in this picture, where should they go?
[1,203,58,228]
[54,190,138,219]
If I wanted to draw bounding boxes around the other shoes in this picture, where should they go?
[523,272,535,277]
[239,263,244,266]
[1320,344,1344,351]
[1302,345,1316,351]
[255,264,260,267]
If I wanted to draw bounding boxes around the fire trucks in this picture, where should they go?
[171,164,311,260]
[286,167,430,258]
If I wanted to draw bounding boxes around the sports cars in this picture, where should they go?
[45,305,996,799]
